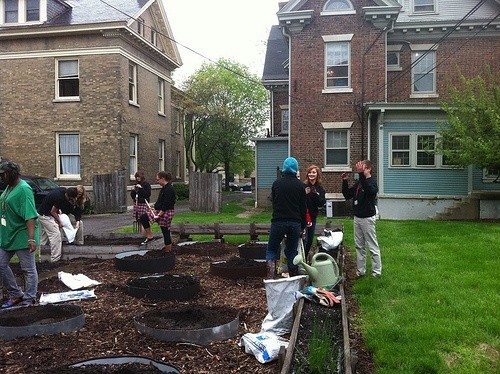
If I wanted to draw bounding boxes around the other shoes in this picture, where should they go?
[77,244,84,246]
[66,241,77,245]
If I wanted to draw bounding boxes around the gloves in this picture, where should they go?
[292,286,342,307]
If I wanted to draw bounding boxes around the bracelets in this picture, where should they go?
[27,240,36,243]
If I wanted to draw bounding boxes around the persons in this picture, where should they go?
[341,159,382,278]
[34,185,88,266]
[266,157,309,280]
[130,171,156,245]
[0,160,40,309]
[278,165,326,279]
[149,172,177,254]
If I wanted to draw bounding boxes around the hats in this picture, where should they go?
[0,161,20,174]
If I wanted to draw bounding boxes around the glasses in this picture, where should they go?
[157,178,161,182]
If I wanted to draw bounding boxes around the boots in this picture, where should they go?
[288,267,298,276]
[266,259,276,279]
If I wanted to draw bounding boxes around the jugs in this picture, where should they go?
[293,252,340,289]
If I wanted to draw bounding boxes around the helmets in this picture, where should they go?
[282,157,298,174]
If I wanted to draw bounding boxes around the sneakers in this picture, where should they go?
[2,294,24,307]
[52,260,68,267]
[350,273,364,280]
[141,237,155,245]
